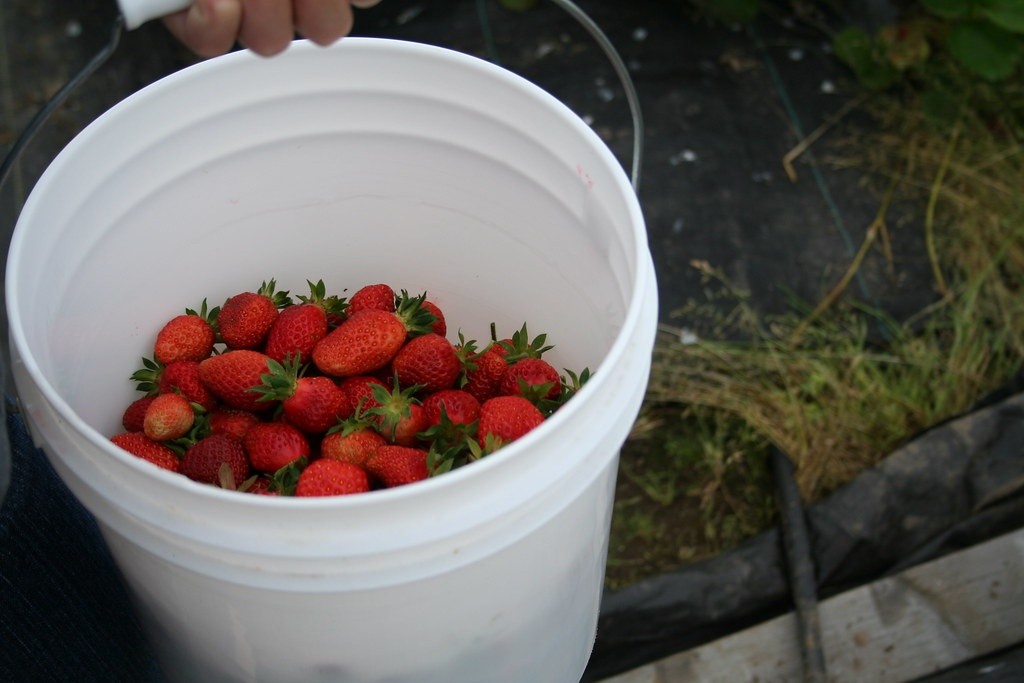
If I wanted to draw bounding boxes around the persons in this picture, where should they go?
[118,0,382,56]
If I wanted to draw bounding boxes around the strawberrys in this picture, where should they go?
[110,277,597,495]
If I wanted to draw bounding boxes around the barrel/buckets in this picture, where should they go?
[6,36,656,682]
